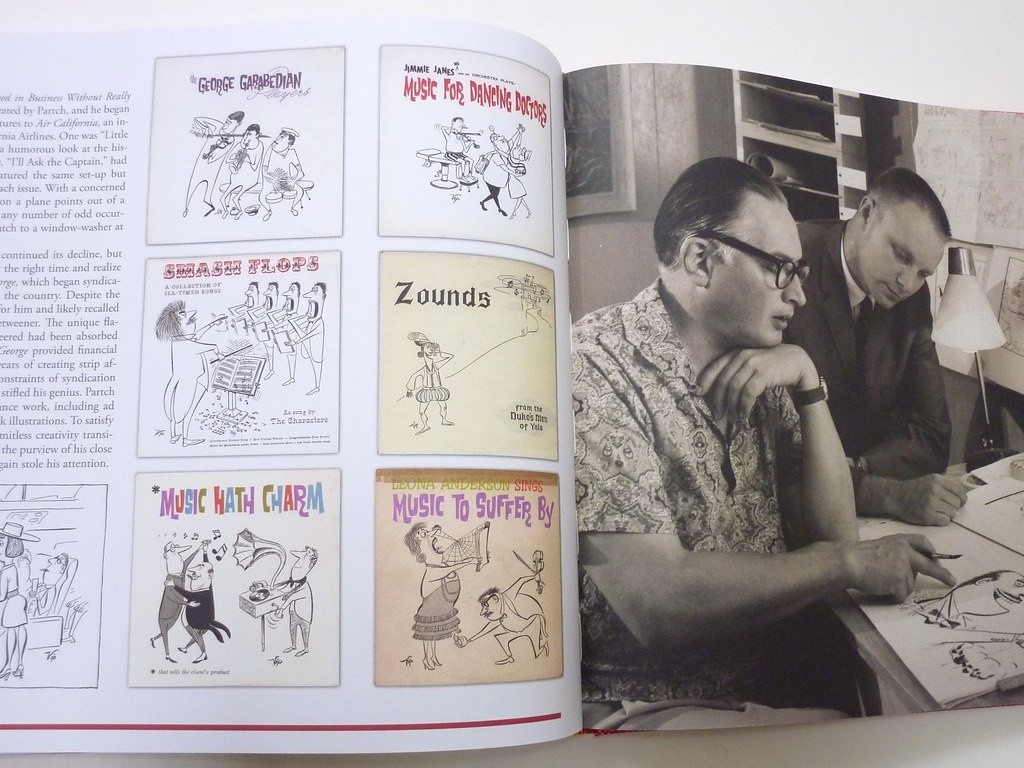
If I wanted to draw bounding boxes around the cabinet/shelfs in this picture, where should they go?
[730,70,868,227]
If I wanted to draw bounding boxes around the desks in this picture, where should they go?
[792,461,1024,714]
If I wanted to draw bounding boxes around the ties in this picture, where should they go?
[854,297,873,360]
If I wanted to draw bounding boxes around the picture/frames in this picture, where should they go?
[562,64,636,220]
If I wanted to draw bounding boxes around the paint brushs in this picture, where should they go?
[919,546,968,565]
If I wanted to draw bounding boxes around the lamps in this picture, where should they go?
[931,247,1008,425]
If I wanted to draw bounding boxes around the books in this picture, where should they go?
[0,17,1024,754]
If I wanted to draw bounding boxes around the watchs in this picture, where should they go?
[789,377,828,409]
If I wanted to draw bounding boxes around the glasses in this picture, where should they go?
[678,225,810,290]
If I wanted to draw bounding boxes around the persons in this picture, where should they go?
[570,154,967,731]
